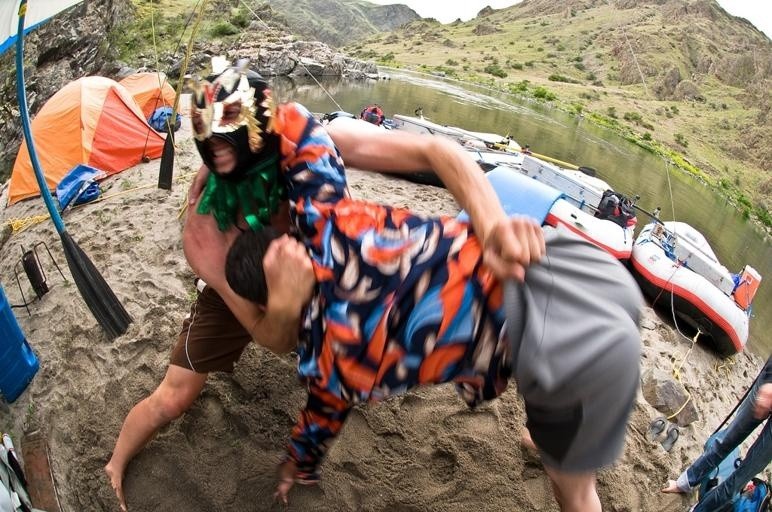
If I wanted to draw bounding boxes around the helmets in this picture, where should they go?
[185,68,281,177]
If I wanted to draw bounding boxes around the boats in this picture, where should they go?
[631,220,752,358]
[326,110,528,179]
[490,160,635,262]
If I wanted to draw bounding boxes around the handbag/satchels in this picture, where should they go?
[151,106,181,133]
[719,460,768,510]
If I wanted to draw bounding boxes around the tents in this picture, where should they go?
[116,72,181,117]
[6,76,171,207]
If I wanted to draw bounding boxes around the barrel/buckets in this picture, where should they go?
[733,265,762,312]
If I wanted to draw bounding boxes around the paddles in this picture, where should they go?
[158,1,206,190]
[484,140,595,176]
[16,0,134,343]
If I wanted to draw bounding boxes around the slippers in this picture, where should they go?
[643,417,668,443]
[661,422,680,452]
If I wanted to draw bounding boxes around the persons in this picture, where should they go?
[103,74,549,510]
[660,354,772,511]
[225,200,645,512]
